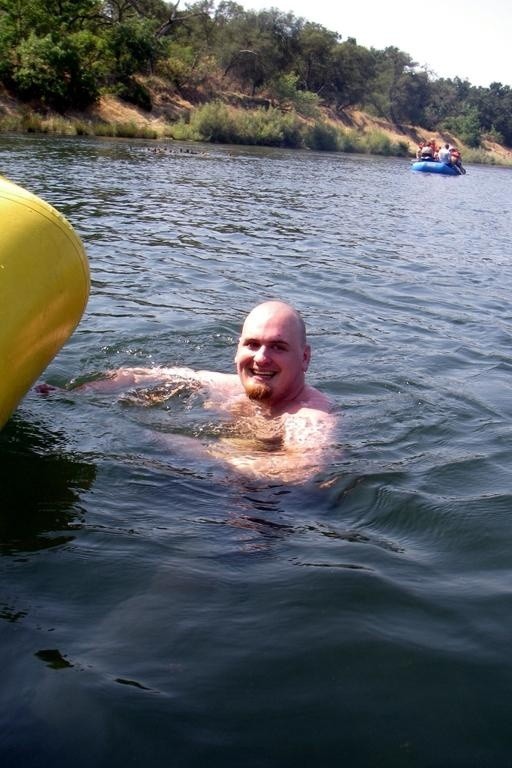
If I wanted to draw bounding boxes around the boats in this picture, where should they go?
[0,173,92,432]
[412,161,461,175]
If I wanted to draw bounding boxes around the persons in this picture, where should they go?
[32,299,336,488]
[416,137,463,166]
